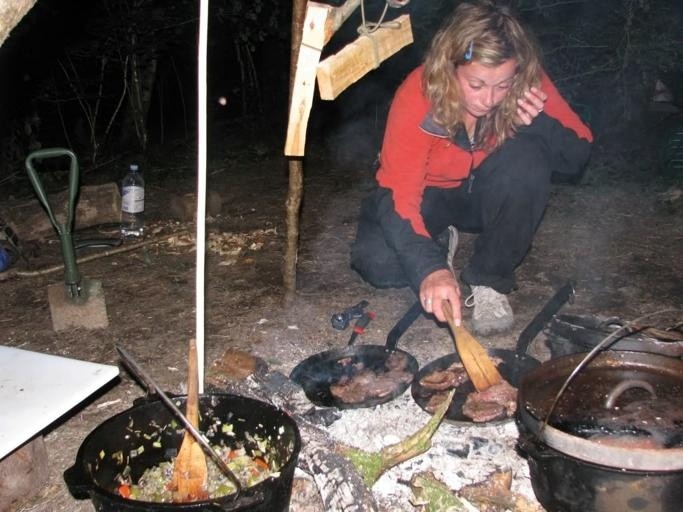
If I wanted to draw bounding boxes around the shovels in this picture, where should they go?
[24,147,109,333]
[171,337,209,504]
[440,299,504,393]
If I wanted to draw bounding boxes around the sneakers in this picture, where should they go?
[465,284,515,336]
[444,223,458,277]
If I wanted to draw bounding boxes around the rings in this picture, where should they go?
[422,298,434,306]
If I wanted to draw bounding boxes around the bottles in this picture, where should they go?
[120,165,145,237]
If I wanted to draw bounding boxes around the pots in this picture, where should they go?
[412,279,576,424]
[63,392,302,511]
[548,314,682,365]
[290,300,422,407]
[513,351,683,512]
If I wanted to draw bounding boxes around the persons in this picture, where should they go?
[347,0,595,326]
